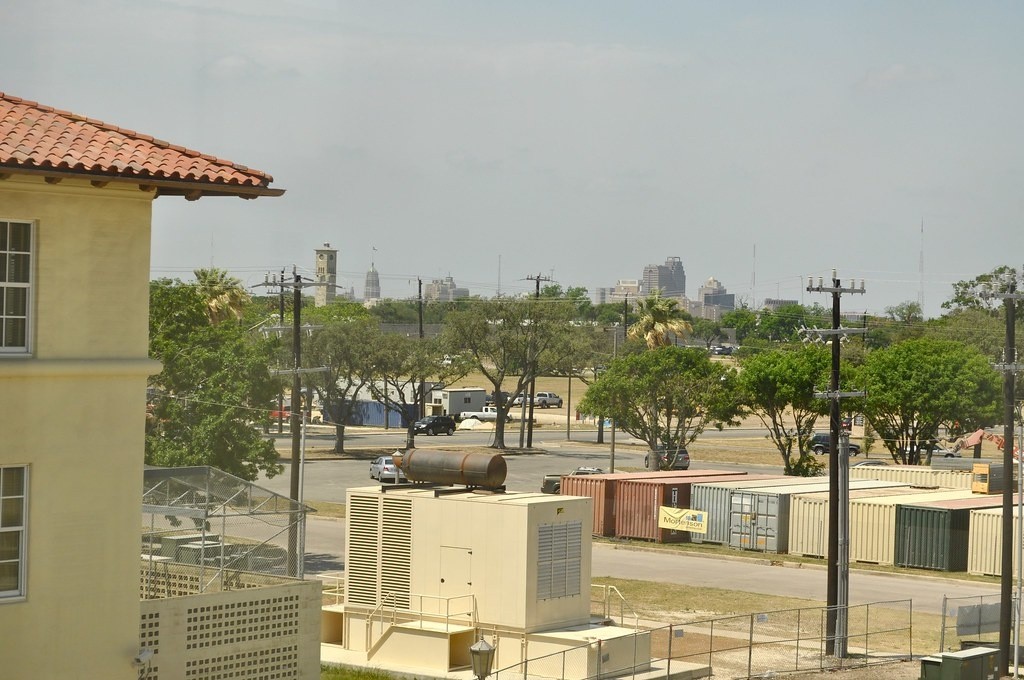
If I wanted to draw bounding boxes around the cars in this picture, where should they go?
[850,461,888,467]
[369,456,409,482]
[594,363,610,369]
[486,314,621,328]
[901,443,961,459]
[703,343,736,355]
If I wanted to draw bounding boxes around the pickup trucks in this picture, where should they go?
[527,392,563,408]
[541,469,607,495]
[506,392,532,407]
[460,406,513,423]
[486,391,510,405]
[269,406,310,423]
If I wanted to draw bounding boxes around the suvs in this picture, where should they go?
[807,433,860,457]
[412,415,456,436]
[645,443,691,469]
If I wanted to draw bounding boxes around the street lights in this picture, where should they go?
[296,384,314,578]
